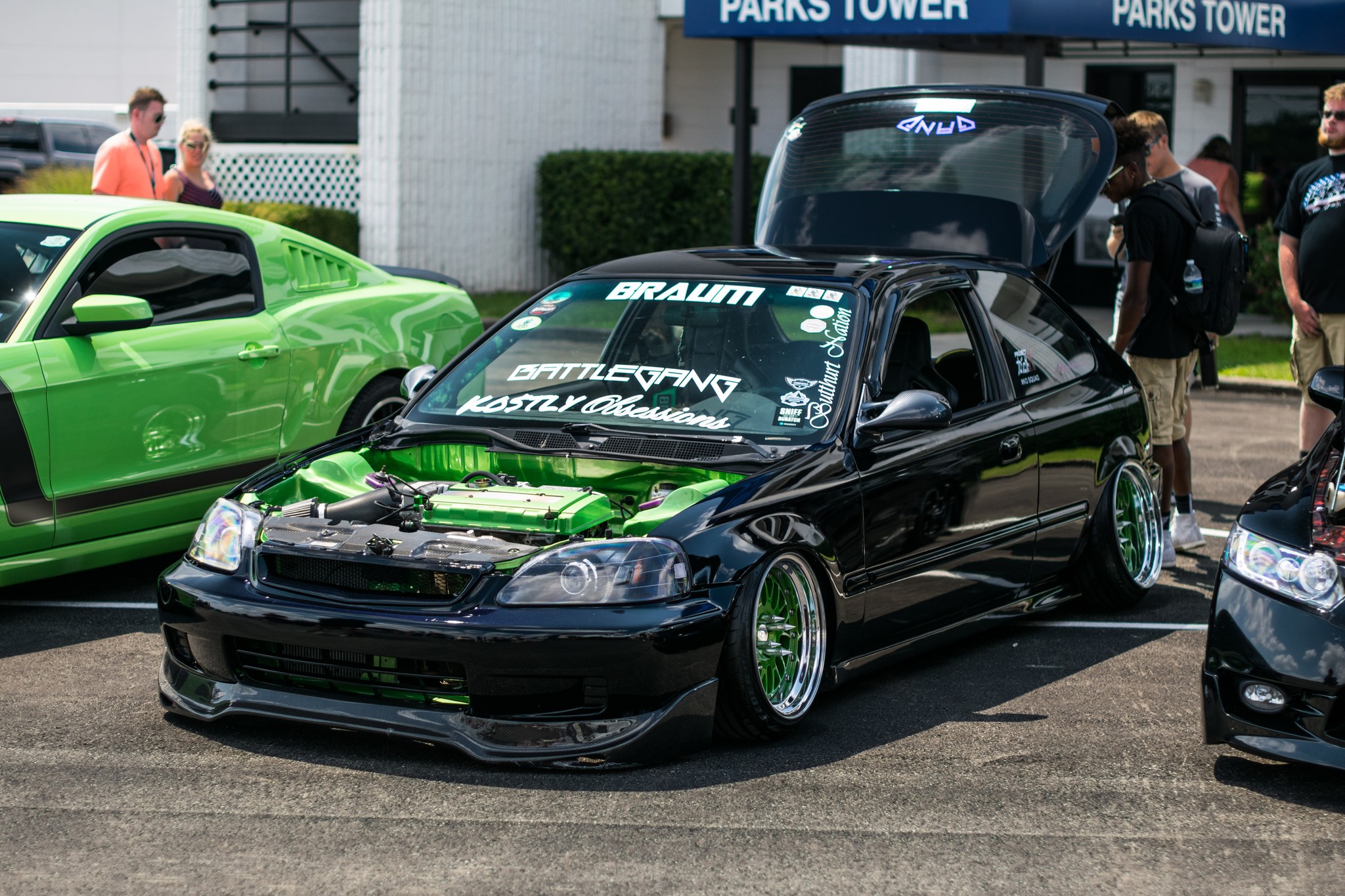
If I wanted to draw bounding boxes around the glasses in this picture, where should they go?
[1322,109,1345,121]
[1103,163,1128,191]
[185,141,209,152]
[143,113,167,124]
[1143,136,1163,157]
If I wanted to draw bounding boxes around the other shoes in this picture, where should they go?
[1170,509,1206,551]
[1159,529,1177,569]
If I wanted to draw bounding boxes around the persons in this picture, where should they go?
[91,88,167,200]
[163,122,223,209]
[1106,110,1246,504]
[1273,83,1345,459]
[1102,117,1207,569]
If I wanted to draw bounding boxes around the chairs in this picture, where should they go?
[679,307,773,402]
[886,317,960,411]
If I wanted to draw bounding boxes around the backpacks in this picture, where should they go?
[1139,179,1252,336]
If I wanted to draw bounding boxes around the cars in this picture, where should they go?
[1195,367,1344,776]
[151,79,1176,779]
[2,115,134,171]
[0,188,489,597]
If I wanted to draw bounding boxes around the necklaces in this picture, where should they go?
[1141,179,1156,188]
